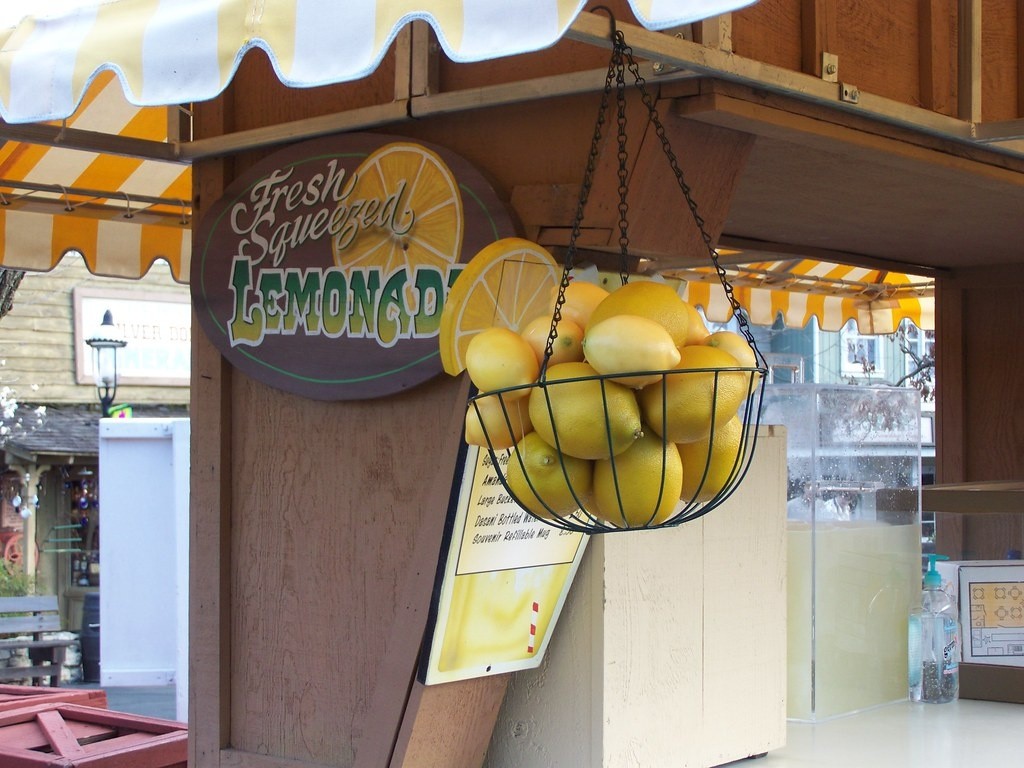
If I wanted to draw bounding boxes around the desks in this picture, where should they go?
[876,479,1024,526]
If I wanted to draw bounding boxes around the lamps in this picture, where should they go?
[86,307,127,417]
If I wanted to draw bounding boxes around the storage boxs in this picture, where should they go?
[82,592,100,636]
[747,382,921,726]
[80,636,100,682]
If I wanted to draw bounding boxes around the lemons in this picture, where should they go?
[464,282,760,525]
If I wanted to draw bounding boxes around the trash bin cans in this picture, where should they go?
[80,593,101,682]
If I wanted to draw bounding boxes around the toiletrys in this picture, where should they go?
[905,553,961,705]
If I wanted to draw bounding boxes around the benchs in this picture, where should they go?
[0,594,80,688]
